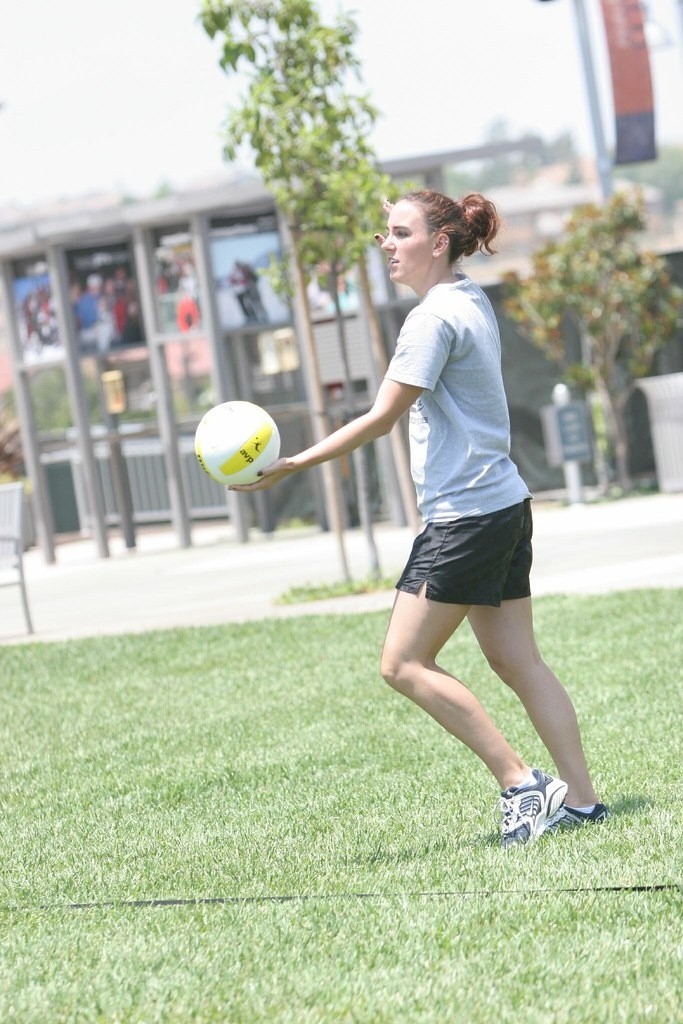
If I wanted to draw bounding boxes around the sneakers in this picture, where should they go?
[494,769,568,850]
[541,801,609,835]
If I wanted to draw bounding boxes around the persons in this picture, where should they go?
[155,249,195,291]
[22,285,59,346]
[71,267,141,353]
[228,192,605,845]
[230,262,267,324]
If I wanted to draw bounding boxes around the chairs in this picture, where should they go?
[0,484,35,635]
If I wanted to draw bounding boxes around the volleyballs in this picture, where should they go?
[191,398,283,484]
